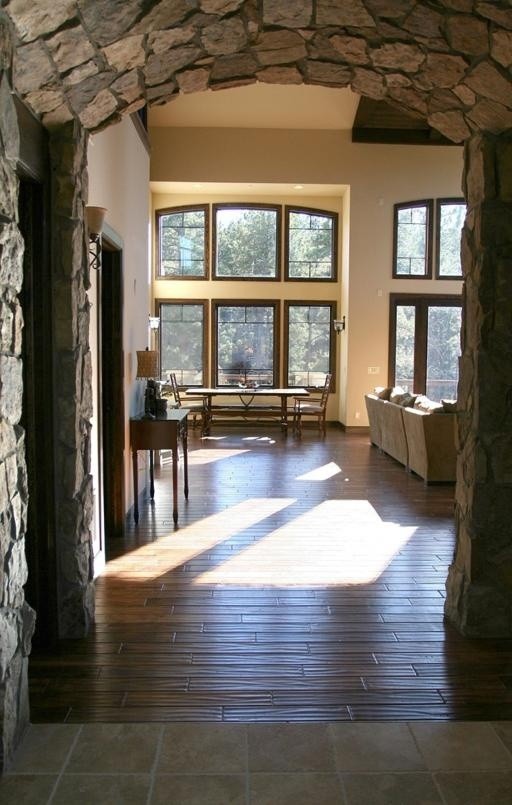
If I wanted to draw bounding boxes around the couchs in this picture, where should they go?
[363,385,459,487]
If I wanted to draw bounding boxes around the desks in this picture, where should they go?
[130,408,191,524]
[183,388,310,437]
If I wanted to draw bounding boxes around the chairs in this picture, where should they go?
[170,373,208,433]
[292,373,334,441]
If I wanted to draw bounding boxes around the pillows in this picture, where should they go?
[373,387,458,414]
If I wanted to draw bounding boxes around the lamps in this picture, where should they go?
[333,315,345,335]
[85,205,109,270]
[134,347,161,420]
[149,313,160,336]
